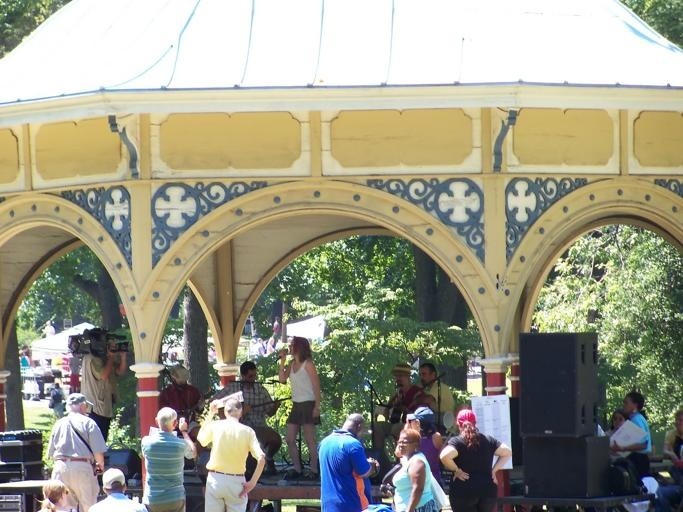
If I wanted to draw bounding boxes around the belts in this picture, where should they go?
[58,458,89,461]
[210,469,245,477]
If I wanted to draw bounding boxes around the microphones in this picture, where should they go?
[269,380,287,384]
[272,349,287,365]
[375,404,393,409]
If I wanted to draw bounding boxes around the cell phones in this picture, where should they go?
[179,417,186,424]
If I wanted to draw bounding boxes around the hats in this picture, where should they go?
[390,364,411,373]
[406,407,435,425]
[102,468,126,489]
[458,409,476,427]
[66,393,94,405]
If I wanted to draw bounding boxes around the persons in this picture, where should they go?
[0,330,681,492]
[315,413,381,512]
[40,480,83,512]
[437,408,512,512]
[379,427,441,512]
[195,397,265,512]
[139,406,198,512]
[86,468,147,512]
[45,393,108,511]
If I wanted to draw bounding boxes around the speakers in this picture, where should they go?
[518,435,609,498]
[196,449,256,483]
[97,449,141,486]
[518,331,599,437]
[363,448,392,486]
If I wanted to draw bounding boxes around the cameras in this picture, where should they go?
[379,484,394,496]
